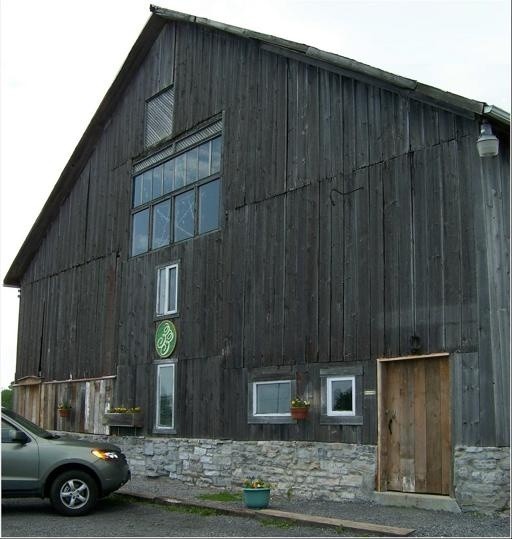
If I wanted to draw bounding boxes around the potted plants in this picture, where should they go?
[58,403,71,418]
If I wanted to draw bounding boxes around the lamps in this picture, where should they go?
[475,116,501,159]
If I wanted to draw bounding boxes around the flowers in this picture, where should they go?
[243,475,267,489]
[287,396,311,407]
[111,406,139,414]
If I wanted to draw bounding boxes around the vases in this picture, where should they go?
[242,487,271,509]
[290,405,308,420]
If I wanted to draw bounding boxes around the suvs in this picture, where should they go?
[0,404,131,516]
